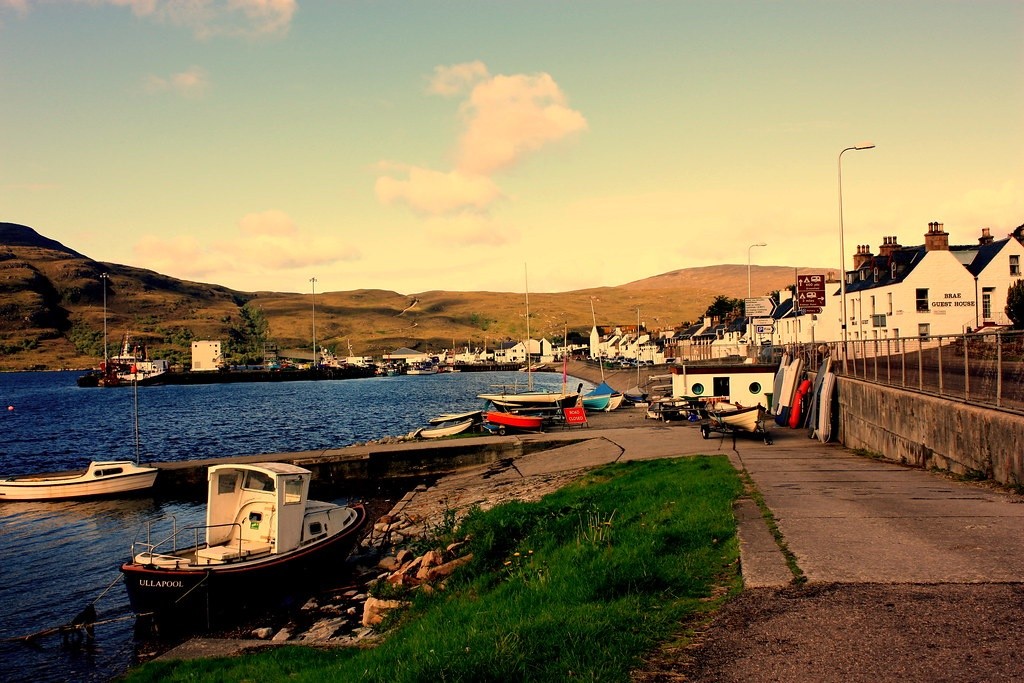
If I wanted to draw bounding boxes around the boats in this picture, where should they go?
[767,352,835,444]
[406,362,437,375]
[707,402,767,434]
[414,419,475,438]
[428,409,484,424]
[0,458,159,502]
[519,363,546,372]
[118,459,371,628]
[486,409,545,428]
[580,392,612,411]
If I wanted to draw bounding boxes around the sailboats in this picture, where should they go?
[476,263,579,414]
[623,309,649,403]
[582,300,624,410]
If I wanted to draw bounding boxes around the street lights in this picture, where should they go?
[745,241,768,345]
[310,276,317,366]
[838,141,879,377]
[100,270,111,372]
